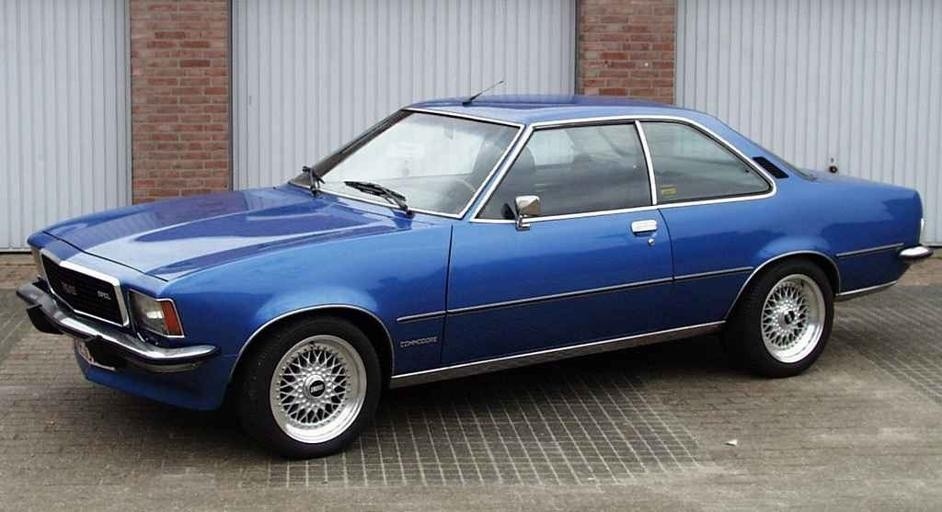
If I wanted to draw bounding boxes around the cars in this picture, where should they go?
[15,79,934,462]
